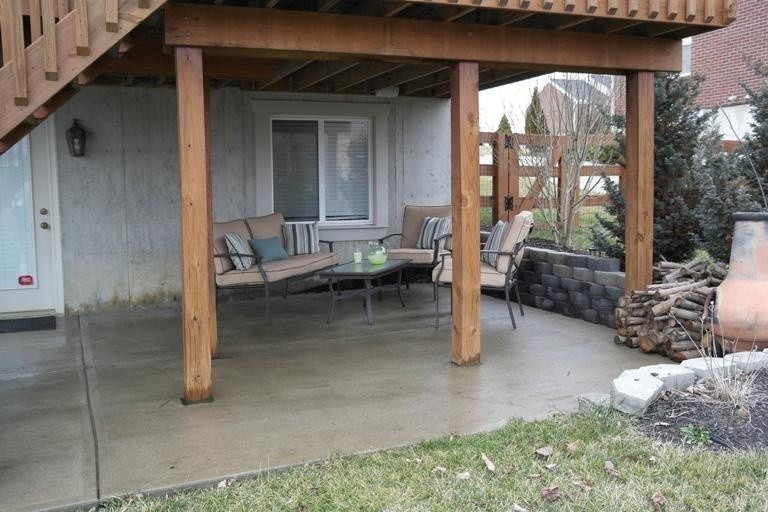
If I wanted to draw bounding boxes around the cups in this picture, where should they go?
[353,247,363,264]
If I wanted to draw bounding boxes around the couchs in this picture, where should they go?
[212,212,342,327]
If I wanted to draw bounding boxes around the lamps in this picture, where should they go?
[64,118,87,157]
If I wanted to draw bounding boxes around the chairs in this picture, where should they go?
[379,205,535,330]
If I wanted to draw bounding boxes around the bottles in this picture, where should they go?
[366,242,388,265]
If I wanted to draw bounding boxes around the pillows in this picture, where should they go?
[223,220,321,271]
